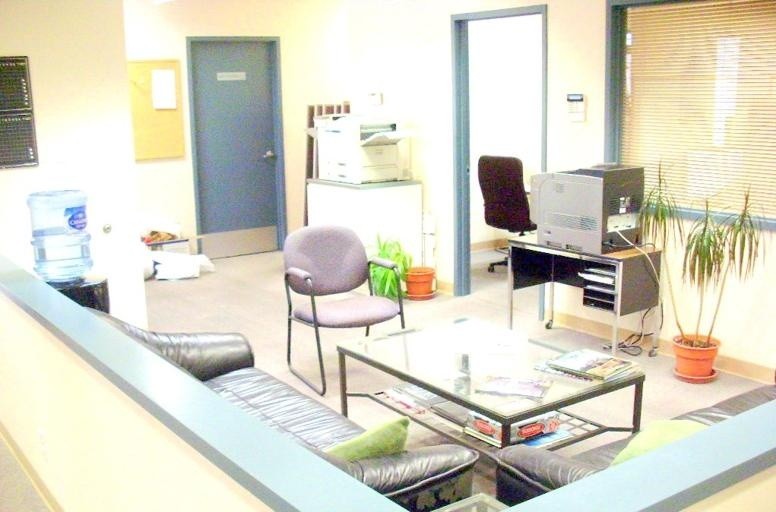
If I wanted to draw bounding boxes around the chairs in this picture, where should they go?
[282,225,405,395]
[478,154,538,272]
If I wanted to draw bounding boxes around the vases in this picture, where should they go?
[672,334,721,383]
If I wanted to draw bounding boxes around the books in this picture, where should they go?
[386,366,561,448]
[546,347,639,383]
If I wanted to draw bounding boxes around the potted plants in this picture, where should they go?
[370,237,438,300]
[638,160,771,384]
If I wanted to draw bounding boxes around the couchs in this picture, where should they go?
[495,382,774,509]
[85,306,479,512]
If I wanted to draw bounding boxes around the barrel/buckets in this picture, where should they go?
[27,188,93,290]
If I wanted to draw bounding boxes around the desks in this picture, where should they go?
[506,234,662,358]
[336,314,646,471]
